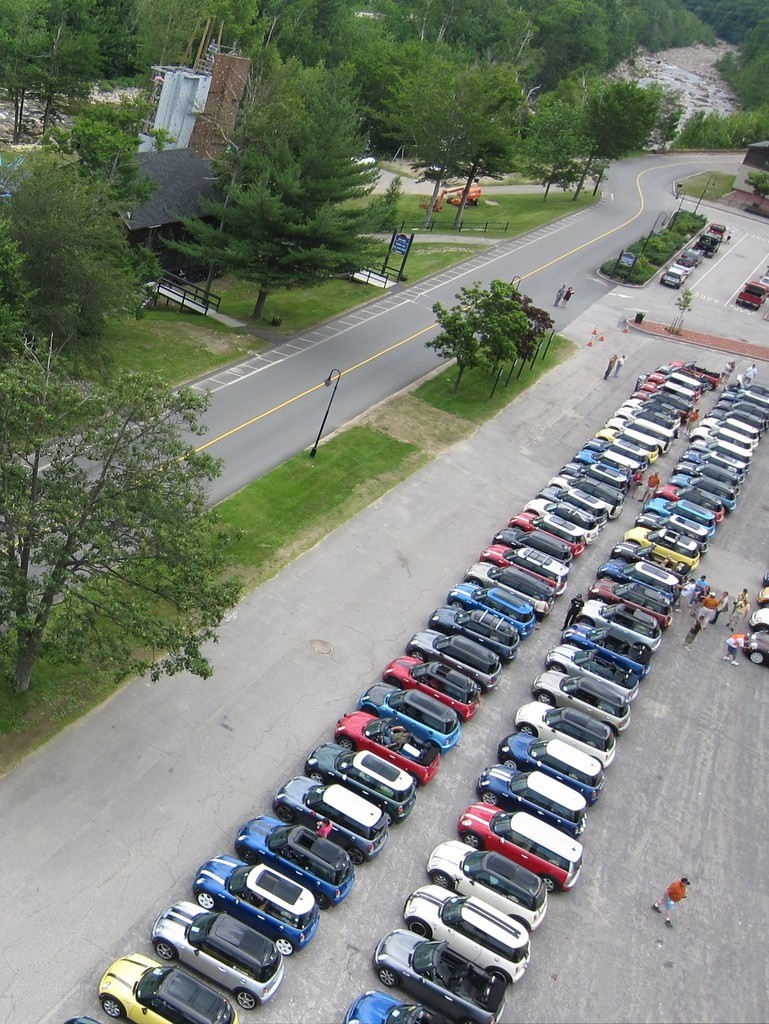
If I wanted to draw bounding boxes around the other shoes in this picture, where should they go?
[709,621,715,624]
[664,920,673,927]
[722,656,730,660]
[535,627,540,630]
[651,905,661,913]
[631,495,635,500]
[638,499,644,502]
[561,627,567,631]
[675,609,681,613]
[726,623,731,626]
[684,645,691,652]
[688,604,691,607]
[731,660,739,666]
[731,628,734,632]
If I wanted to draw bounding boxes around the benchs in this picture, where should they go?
[613,667,633,684]
[455,976,496,1004]
[627,646,645,661]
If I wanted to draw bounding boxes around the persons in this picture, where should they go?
[651,877,691,927]
[534,595,548,630]
[670,410,680,439]
[744,363,757,384]
[631,470,644,499]
[724,227,734,244]
[383,725,410,748]
[613,355,625,377]
[561,594,585,631]
[636,543,657,560]
[638,472,660,504]
[619,465,632,494]
[560,287,574,309]
[726,588,751,632]
[603,355,618,381]
[315,817,332,838]
[722,632,750,665]
[683,615,705,651]
[553,285,566,306]
[675,575,729,625]
[683,408,700,436]
[720,360,736,383]
[588,582,599,600]
[177,265,187,279]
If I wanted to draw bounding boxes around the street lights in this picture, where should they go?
[309,369,341,458]
[623,173,721,285]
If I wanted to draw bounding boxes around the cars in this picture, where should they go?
[64,363,769,1024]
[736,266,769,311]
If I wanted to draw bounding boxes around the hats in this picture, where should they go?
[539,595,544,599]
[710,592,717,597]
[577,594,582,598]
[689,579,695,583]
[681,877,691,885]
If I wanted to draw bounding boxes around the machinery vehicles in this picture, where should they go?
[418,183,481,212]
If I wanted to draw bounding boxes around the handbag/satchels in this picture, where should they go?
[720,600,728,612]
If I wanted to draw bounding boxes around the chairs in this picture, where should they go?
[436,962,470,986]
[605,642,627,653]
[599,662,614,676]
[568,722,592,741]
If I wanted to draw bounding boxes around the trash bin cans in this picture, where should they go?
[635,311,645,323]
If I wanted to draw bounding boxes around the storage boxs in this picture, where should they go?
[400,743,420,759]
